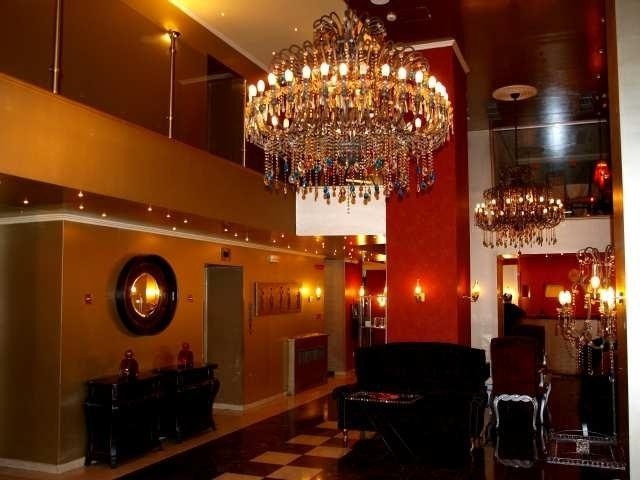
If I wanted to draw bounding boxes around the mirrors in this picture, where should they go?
[115,253,179,336]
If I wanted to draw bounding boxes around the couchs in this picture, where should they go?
[332,341,488,457]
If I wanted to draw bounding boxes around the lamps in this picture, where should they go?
[242,0,455,215]
[474,83,566,255]
[554,242,624,382]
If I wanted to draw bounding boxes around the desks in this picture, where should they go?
[82,361,220,468]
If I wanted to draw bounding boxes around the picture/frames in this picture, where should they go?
[254,280,303,316]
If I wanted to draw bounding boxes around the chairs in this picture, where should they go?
[490,336,551,430]
